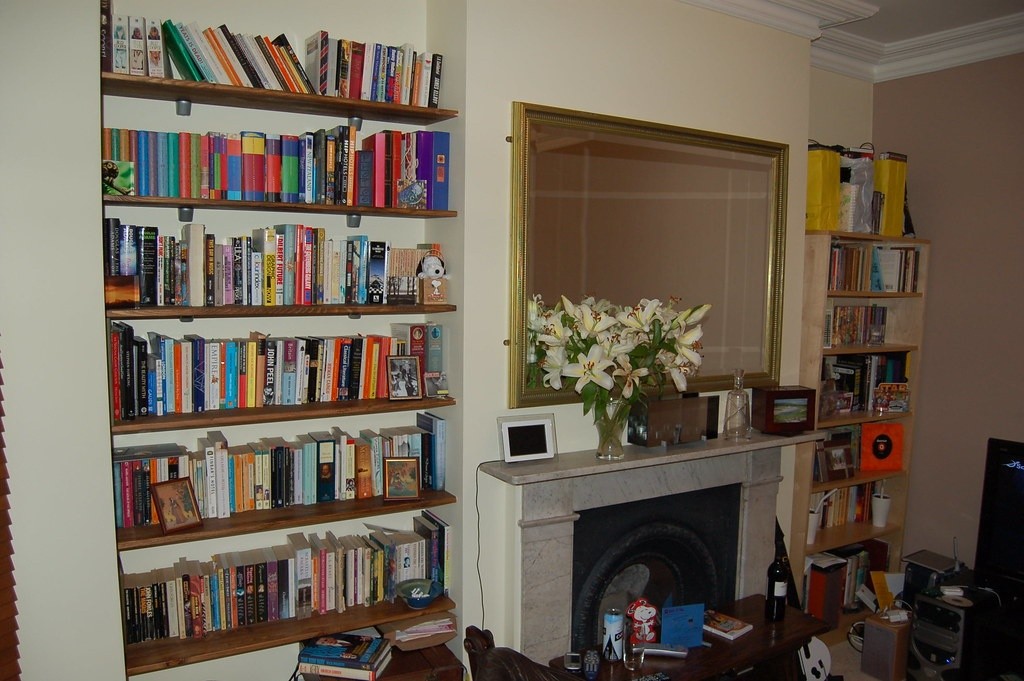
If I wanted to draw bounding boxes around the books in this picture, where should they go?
[113,19,444,108]
[809,481,883,531]
[114,413,446,528]
[821,353,910,418]
[824,298,888,349]
[299,633,392,681]
[105,218,443,311]
[102,126,451,211]
[832,245,920,293]
[111,322,444,419]
[121,508,449,646]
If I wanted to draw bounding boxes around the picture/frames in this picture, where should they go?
[383,456,423,502]
[150,476,205,535]
[816,439,854,484]
[497,413,560,468]
[386,354,423,402]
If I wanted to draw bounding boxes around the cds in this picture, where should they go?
[941,594,973,606]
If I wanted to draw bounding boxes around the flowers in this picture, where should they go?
[534,293,712,451]
[523,293,691,389]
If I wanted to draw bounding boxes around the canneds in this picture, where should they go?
[602,606,625,663]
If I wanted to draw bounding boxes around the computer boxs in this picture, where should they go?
[907,568,1004,681]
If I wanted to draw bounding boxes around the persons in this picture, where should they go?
[144,462,149,471]
[404,557,410,568]
[394,378,399,395]
[314,633,371,658]
[115,25,126,40]
[131,27,142,39]
[320,464,332,479]
[408,364,414,376]
[256,487,263,500]
[116,40,126,68]
[405,373,417,396]
[390,360,400,378]
[170,497,186,524]
[149,51,162,68]
[149,27,160,40]
[398,364,406,379]
[264,490,269,499]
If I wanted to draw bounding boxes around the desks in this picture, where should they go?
[549,593,831,681]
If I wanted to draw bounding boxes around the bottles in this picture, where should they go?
[764,531,788,622]
[722,369,751,438]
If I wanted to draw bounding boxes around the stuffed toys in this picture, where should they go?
[416,254,446,279]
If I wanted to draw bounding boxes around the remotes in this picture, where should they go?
[637,672,670,681]
[583,650,600,680]
[630,642,688,658]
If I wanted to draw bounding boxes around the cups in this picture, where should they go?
[871,494,891,528]
[622,621,645,671]
[806,508,819,545]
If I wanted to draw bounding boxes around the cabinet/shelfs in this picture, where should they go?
[788,229,932,645]
[97,0,458,676]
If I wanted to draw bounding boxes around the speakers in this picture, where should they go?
[975,437,1024,585]
[861,607,913,680]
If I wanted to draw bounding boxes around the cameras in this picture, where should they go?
[563,651,581,672]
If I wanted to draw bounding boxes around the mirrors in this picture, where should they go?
[507,100,790,409]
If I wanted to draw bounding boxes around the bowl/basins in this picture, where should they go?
[395,577,443,610]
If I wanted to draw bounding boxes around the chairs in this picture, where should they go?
[464,625,584,681]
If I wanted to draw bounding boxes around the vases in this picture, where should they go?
[592,397,629,461]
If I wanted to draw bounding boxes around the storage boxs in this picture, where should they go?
[751,384,816,433]
